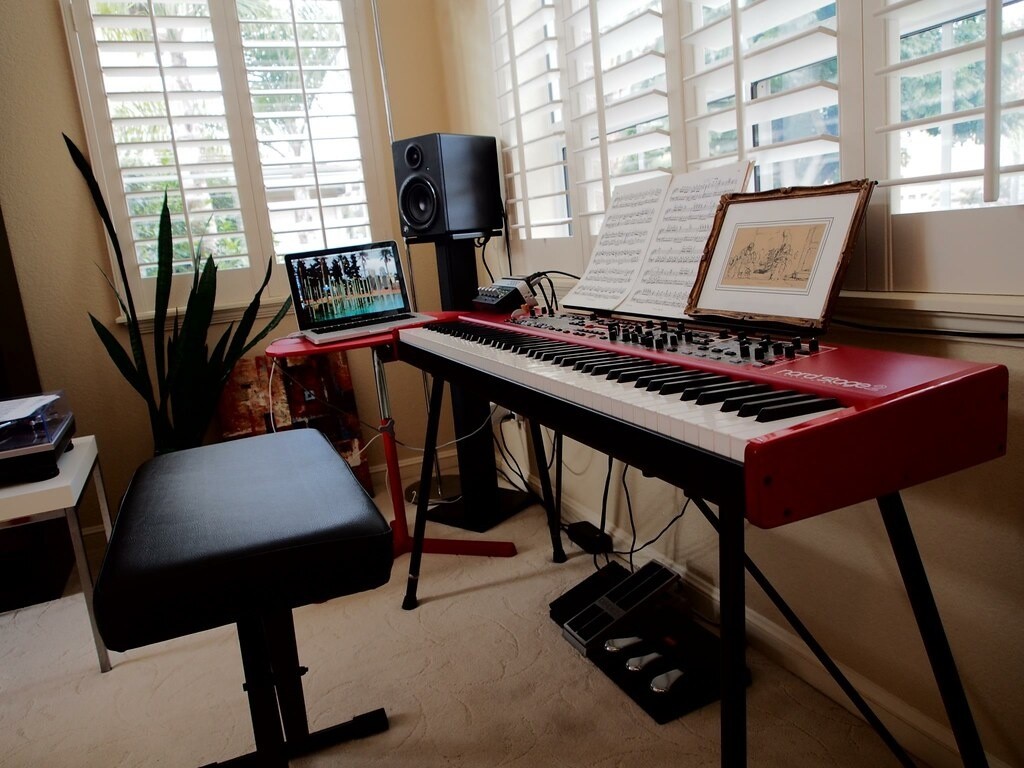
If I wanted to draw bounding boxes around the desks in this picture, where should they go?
[0,434,113,674]
[265,311,517,560]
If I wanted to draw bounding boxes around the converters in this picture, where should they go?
[567,521,613,554]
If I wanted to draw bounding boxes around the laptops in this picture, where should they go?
[284,241,438,345]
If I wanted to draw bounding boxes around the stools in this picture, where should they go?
[91,427,393,768]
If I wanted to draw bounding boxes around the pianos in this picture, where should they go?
[391,307,1010,530]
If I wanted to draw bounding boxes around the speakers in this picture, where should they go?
[391,133,504,237]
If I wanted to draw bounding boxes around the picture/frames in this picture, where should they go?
[683,178,878,331]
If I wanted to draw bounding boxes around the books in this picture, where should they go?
[559,159,756,322]
